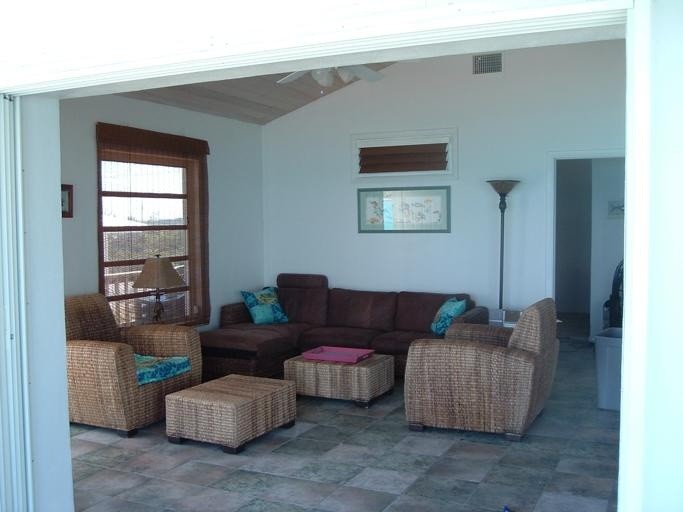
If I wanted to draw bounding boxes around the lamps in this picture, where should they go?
[133,254,186,323]
[487,180,519,308]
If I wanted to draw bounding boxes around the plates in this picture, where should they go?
[301,346,375,364]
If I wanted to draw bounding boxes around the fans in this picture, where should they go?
[276,64,385,88]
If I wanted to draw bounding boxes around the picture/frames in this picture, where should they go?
[61,183,73,218]
[358,186,451,233]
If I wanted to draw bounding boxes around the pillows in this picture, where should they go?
[430,297,466,335]
[238,286,289,324]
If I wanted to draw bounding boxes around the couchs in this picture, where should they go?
[404,298,560,444]
[199,272,490,379]
[63,293,203,438]
